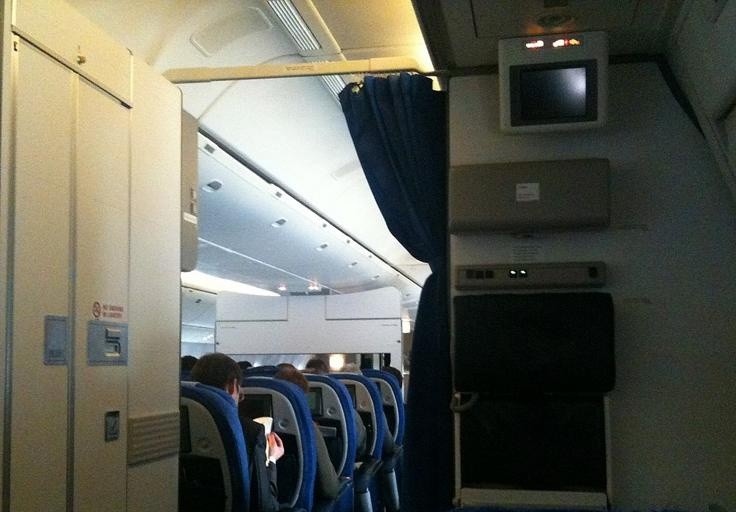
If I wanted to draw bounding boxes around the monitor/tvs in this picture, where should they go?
[496,29,609,134]
[238,384,358,424]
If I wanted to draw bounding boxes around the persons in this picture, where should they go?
[272,367,339,500]
[187,352,285,512]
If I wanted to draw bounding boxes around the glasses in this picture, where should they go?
[238,385,245,403]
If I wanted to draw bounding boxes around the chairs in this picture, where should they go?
[177,366,403,512]
